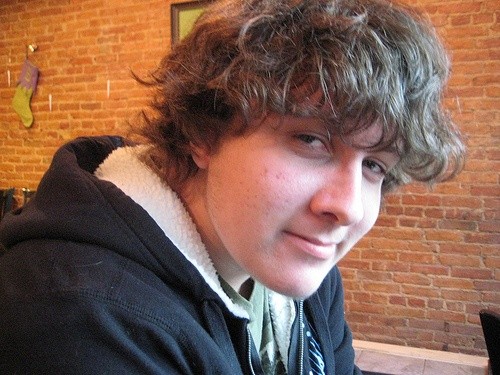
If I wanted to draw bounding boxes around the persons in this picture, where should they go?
[0,1,468,375]
